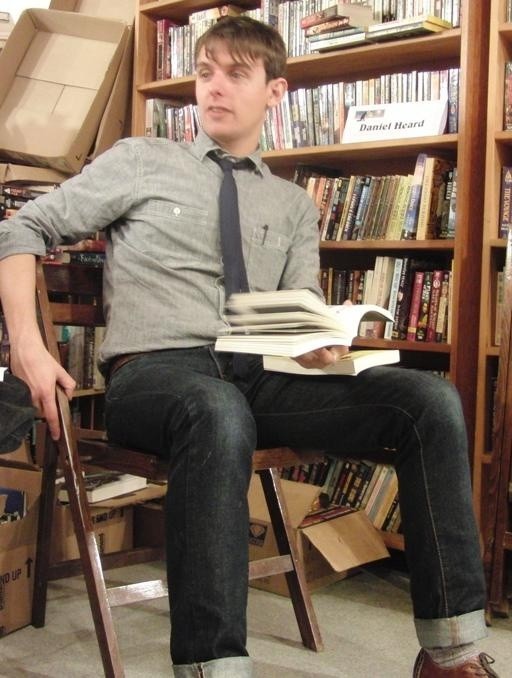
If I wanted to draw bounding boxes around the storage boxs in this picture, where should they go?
[0,0,131,175]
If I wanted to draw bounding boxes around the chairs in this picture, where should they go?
[32,239,322,678]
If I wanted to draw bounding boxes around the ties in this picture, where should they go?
[205,148,254,336]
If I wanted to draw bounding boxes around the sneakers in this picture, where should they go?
[413,648,501,678]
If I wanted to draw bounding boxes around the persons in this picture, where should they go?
[0,17,500,678]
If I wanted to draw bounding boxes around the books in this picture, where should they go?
[318,257,451,343]
[145,98,200,142]
[278,458,401,531]
[54,459,153,507]
[55,326,108,431]
[156,0,461,80]
[292,152,457,241]
[260,69,460,150]
[215,289,400,376]
